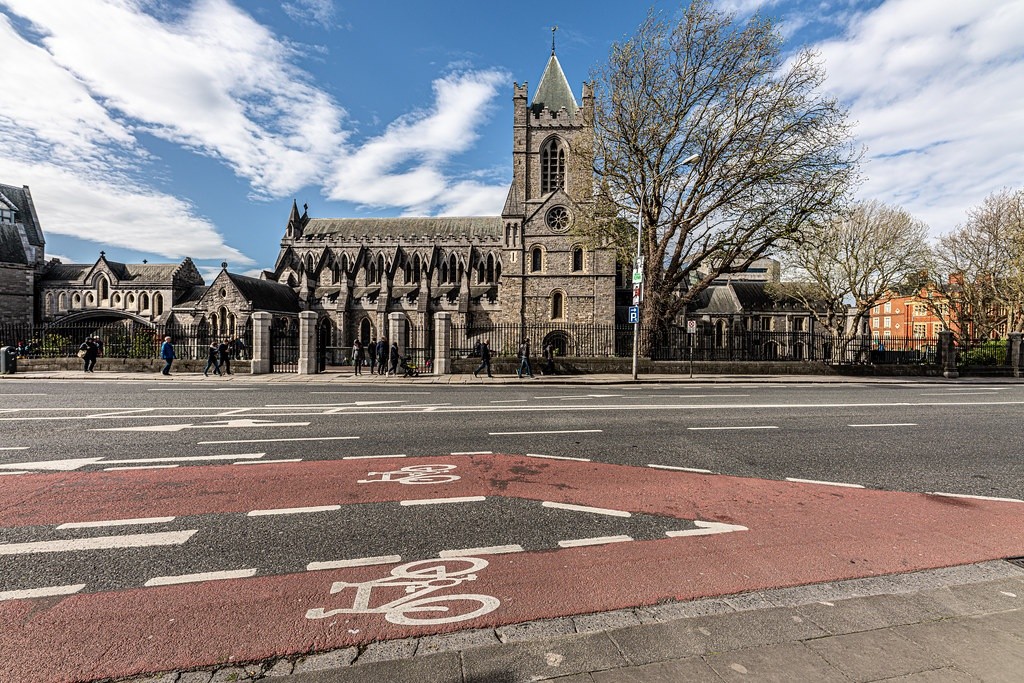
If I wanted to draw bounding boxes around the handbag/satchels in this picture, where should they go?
[77,343,89,358]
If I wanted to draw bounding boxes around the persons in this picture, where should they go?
[204,336,249,377]
[516,338,534,379]
[879,343,885,351]
[160,336,176,375]
[422,356,431,368]
[376,336,389,375]
[386,342,402,377]
[79,336,103,372]
[473,339,493,378]
[351,339,364,376]
[540,342,559,375]
[367,338,377,374]
[15,339,44,359]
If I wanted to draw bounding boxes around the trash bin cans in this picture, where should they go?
[0,346,17,374]
